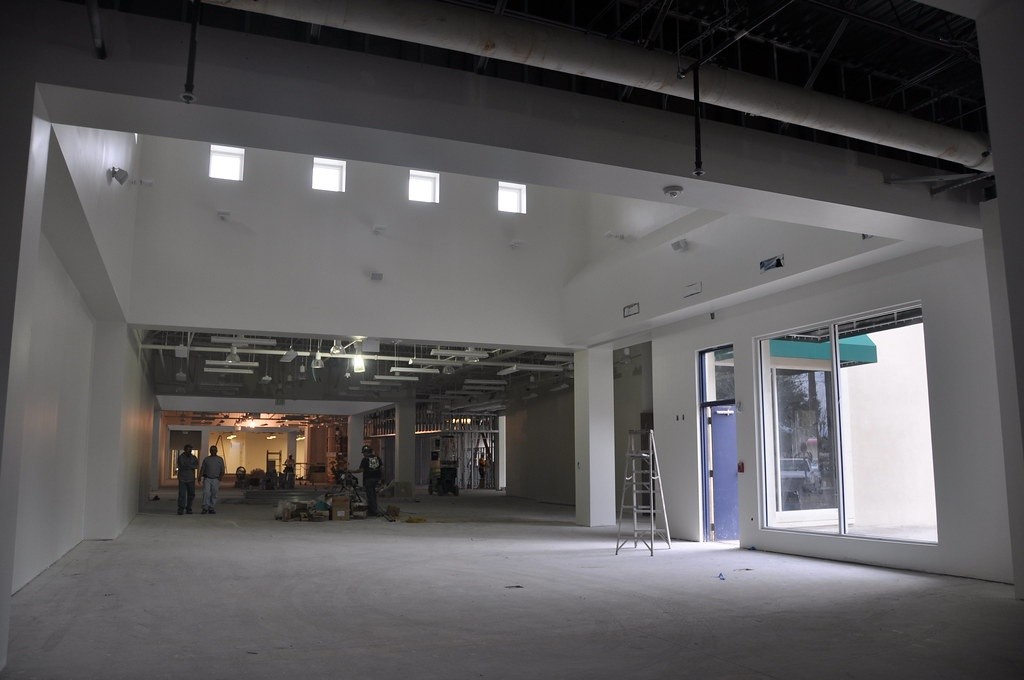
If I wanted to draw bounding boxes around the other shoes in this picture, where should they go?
[209,508,216,515]
[186,509,193,514]
[178,509,184,515]
[200,509,208,514]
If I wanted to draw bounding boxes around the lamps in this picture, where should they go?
[671,239,687,250]
[111,166,127,185]
[175,337,632,413]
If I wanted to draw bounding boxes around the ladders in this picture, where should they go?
[473,433,490,466]
[266,449,281,490]
[616,429,672,557]
[215,435,228,475]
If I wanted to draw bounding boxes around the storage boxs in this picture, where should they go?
[331,495,350,521]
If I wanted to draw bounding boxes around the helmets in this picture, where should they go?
[360,445,373,453]
[288,453,292,456]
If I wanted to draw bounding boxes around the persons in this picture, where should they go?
[285,453,295,473]
[177,444,200,515]
[478,454,485,482]
[198,446,225,514]
[349,445,383,516]
[335,427,342,452]
